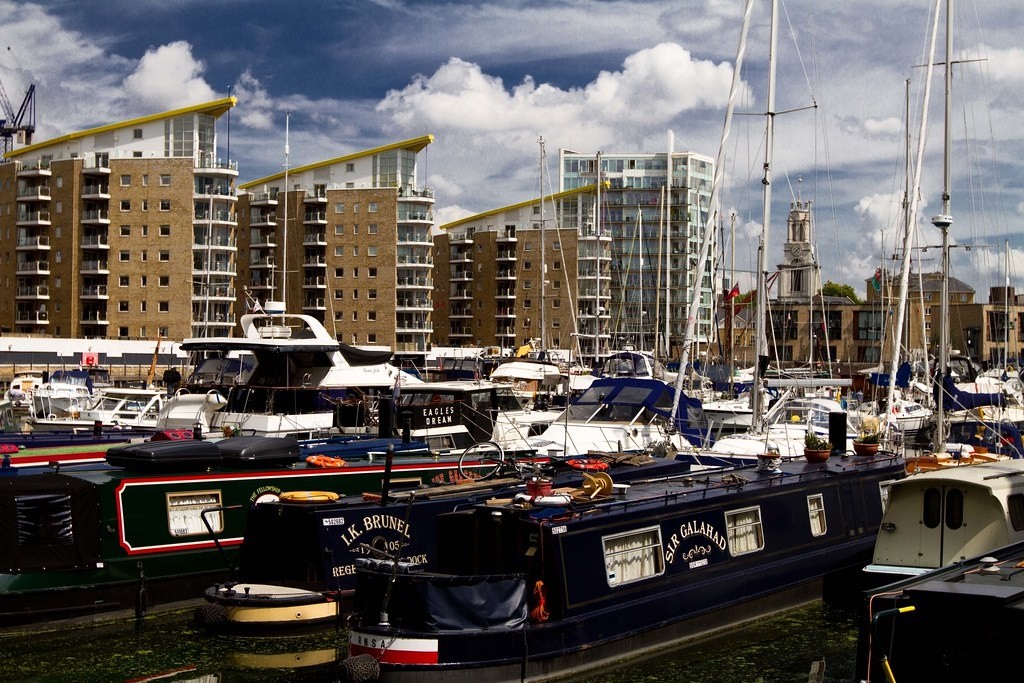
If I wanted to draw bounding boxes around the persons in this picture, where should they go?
[163,366,175,399]
[173,366,181,393]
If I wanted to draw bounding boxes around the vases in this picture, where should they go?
[526,480,553,497]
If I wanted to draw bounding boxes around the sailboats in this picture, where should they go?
[0,0,1024,683]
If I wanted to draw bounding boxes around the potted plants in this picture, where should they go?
[853,434,879,456]
[804,433,833,462]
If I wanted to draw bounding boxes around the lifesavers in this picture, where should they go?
[306,454,344,467]
[279,491,340,503]
[567,459,608,470]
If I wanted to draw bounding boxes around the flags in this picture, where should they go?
[253,298,261,313]
[726,282,740,302]
